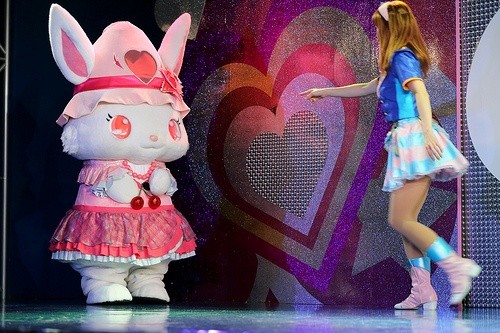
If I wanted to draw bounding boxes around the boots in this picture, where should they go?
[394,256,438,311]
[424,237,482,305]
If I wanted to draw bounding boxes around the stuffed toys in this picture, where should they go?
[47,3,191,306]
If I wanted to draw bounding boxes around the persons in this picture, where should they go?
[299,0,482,310]
